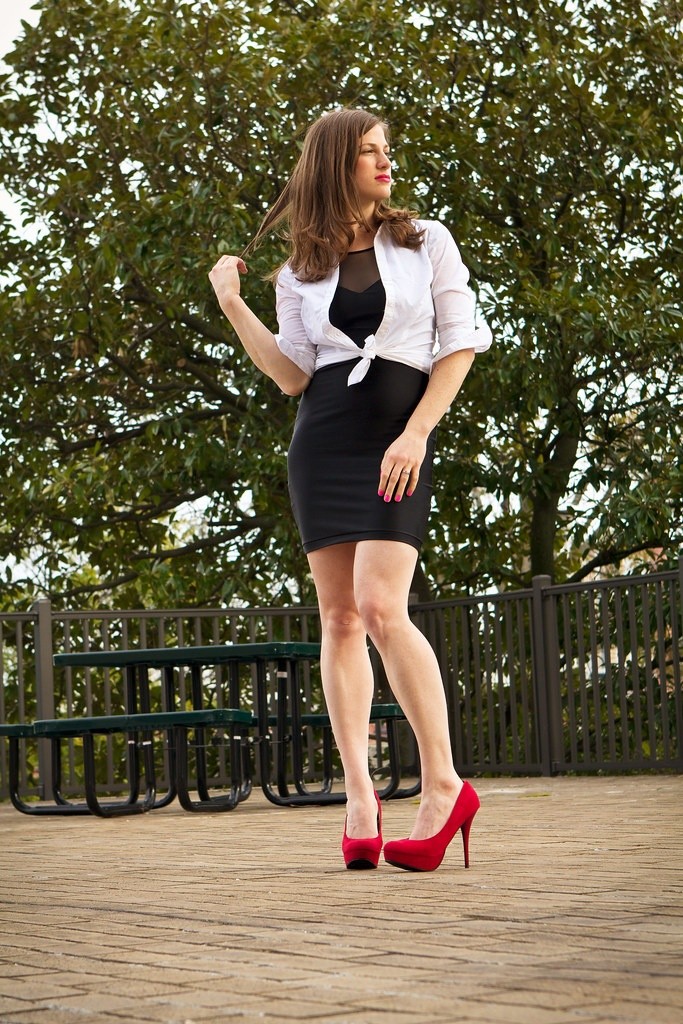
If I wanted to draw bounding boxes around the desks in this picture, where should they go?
[48,640,373,796]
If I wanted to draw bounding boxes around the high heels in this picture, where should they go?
[342,788,383,869]
[383,781,481,871]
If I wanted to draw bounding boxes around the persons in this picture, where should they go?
[208,110,494,870]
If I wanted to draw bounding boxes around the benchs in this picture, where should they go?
[1,701,421,818]
